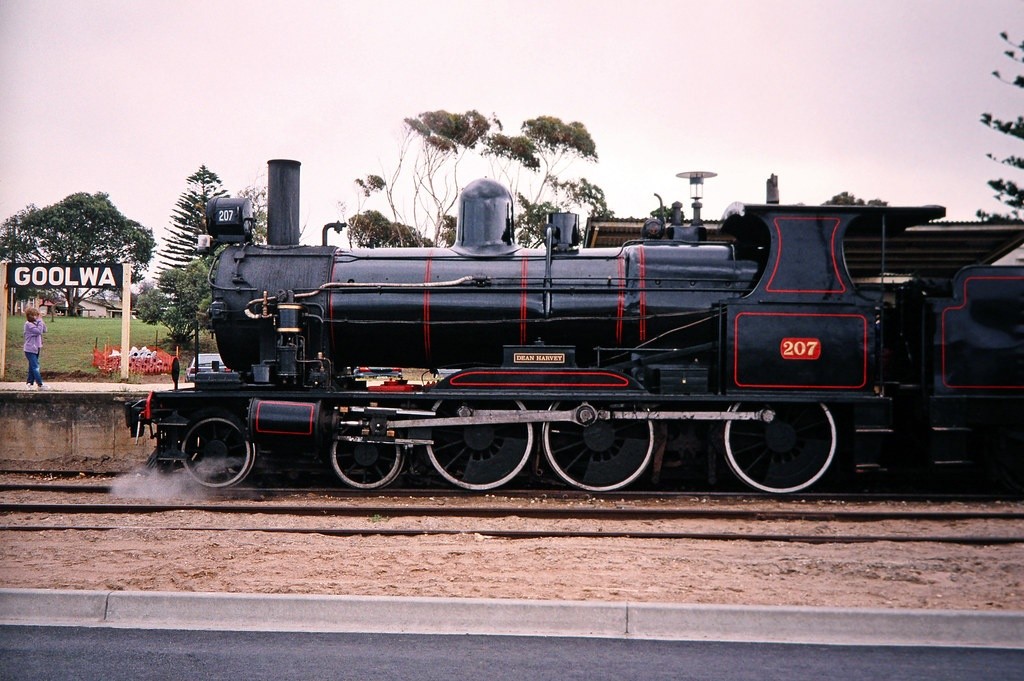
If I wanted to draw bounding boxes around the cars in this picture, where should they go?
[185,353,234,383]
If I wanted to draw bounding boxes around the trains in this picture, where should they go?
[125,159,1024,494]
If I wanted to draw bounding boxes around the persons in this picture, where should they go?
[23,307,48,390]
